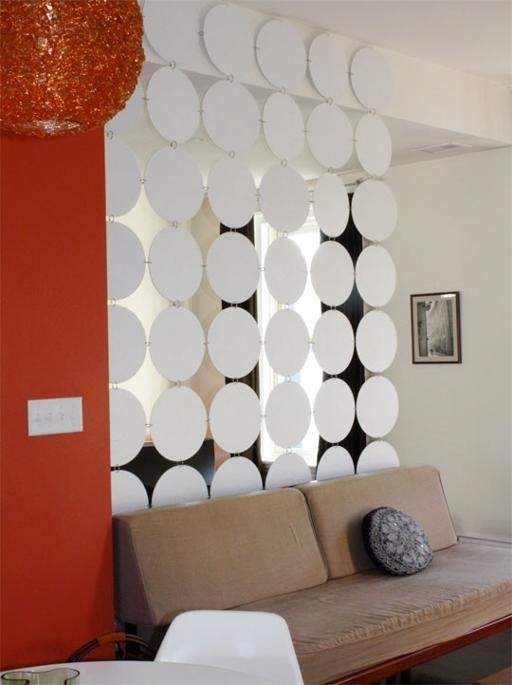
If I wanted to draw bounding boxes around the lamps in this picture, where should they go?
[0,0,145,139]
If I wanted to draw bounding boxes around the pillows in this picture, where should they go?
[358,505,435,576]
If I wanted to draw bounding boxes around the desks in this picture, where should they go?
[111,438,216,508]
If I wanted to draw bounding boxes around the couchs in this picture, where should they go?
[112,466,512,685]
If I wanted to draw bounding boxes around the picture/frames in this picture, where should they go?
[410,290,463,365]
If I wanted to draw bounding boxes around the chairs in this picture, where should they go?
[154,609,304,685]
